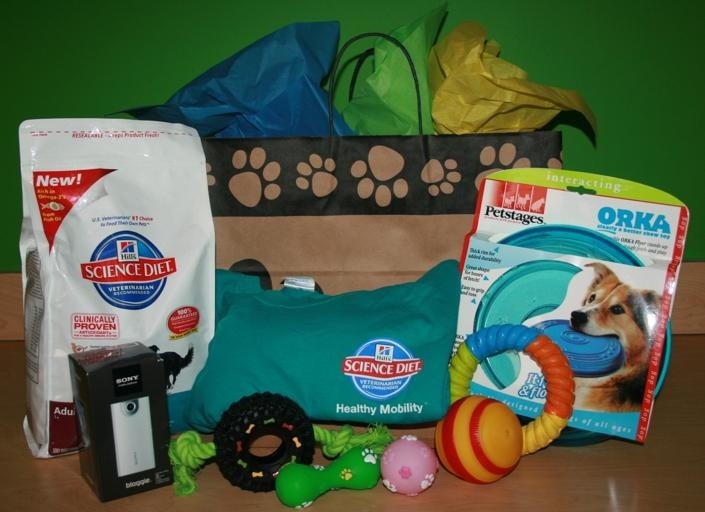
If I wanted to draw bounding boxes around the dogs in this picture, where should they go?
[567,260,664,413]
